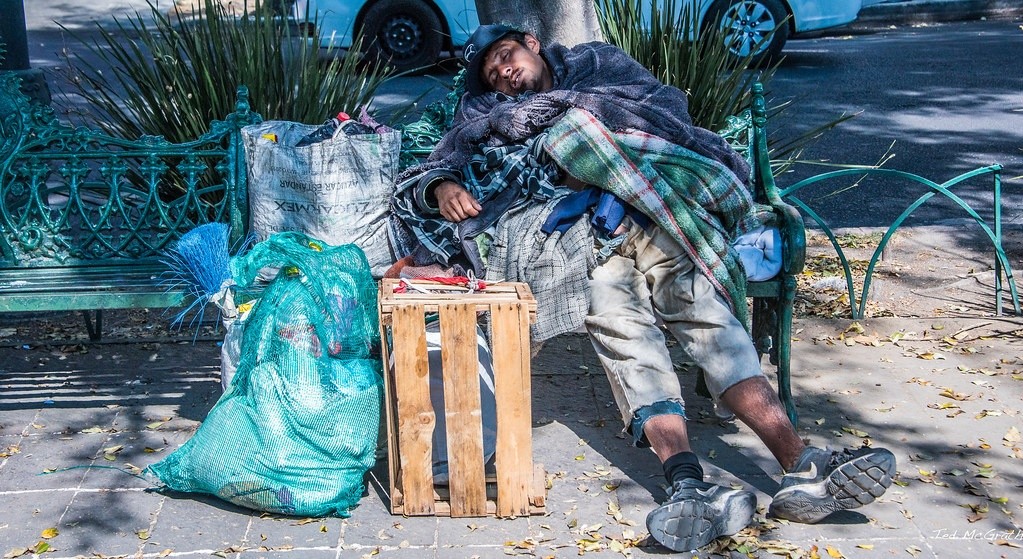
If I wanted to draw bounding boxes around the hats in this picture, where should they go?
[462,26,516,96]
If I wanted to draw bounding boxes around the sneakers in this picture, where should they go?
[646,478,757,551]
[770,446,897,525]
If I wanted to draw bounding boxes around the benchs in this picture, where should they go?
[0,72,807,431]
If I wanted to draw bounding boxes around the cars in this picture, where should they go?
[292,0,863,78]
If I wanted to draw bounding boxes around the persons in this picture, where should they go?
[415,25,897,553]
